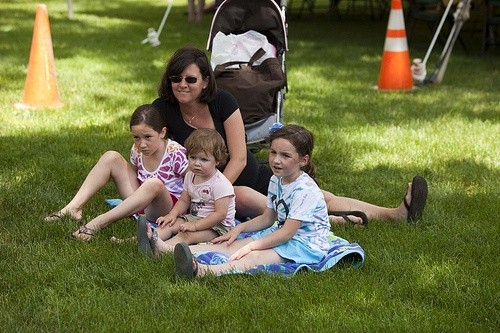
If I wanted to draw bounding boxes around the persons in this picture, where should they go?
[45,104,365,280]
[185,0,217,22]
[147,46,428,225]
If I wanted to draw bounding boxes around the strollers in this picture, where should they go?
[207,1,290,163]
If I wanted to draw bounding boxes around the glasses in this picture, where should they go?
[170,75,202,83]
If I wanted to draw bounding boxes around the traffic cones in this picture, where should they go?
[372,0,418,92]
[10,5,64,109]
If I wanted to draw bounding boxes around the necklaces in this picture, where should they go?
[182,105,203,124]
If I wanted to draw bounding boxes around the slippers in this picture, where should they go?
[327,210,368,226]
[70,226,97,241]
[403,175,427,222]
[136,215,157,255]
[51,211,65,220]
[173,242,197,280]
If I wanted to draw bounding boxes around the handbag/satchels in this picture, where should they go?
[211,47,284,124]
